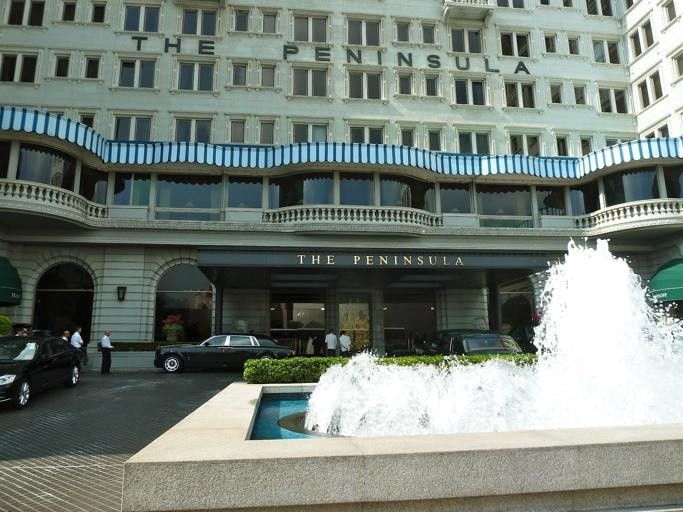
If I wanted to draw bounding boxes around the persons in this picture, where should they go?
[339,330,351,356]
[100,329,112,373]
[61,330,69,341]
[324,329,336,356]
[70,327,83,350]
[14,325,28,336]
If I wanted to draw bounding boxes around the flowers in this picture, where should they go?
[160,314,185,333]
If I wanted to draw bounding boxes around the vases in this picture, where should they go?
[166,331,179,341]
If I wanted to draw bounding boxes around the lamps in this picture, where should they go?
[117,285,127,301]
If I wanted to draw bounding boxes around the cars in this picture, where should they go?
[152,331,298,375]
[423,326,523,355]
[0,331,89,411]
